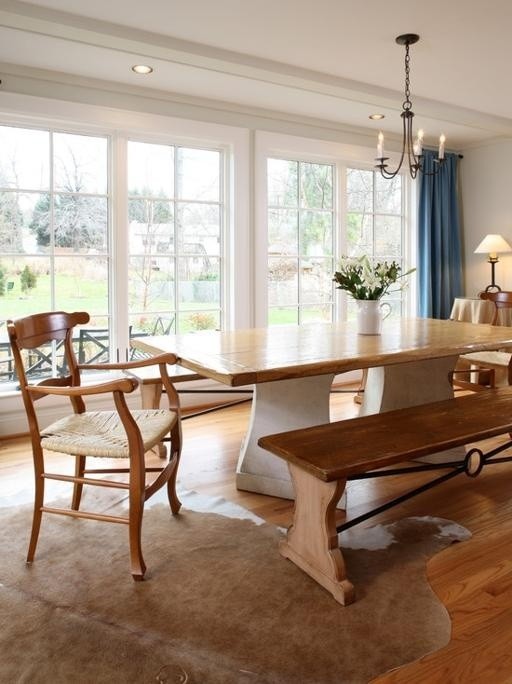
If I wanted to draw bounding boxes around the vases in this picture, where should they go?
[354,300,392,336]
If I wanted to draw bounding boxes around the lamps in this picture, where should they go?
[474,234,512,294]
[373,32,449,180]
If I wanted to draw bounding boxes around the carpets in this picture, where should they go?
[0,444,474,684]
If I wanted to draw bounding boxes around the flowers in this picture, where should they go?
[329,254,416,299]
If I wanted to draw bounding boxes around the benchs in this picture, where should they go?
[257,394,512,605]
[121,361,369,459]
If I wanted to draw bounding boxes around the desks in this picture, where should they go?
[0,328,149,380]
[129,315,512,510]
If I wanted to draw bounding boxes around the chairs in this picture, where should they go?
[28,336,72,379]
[5,310,183,582]
[78,325,133,365]
